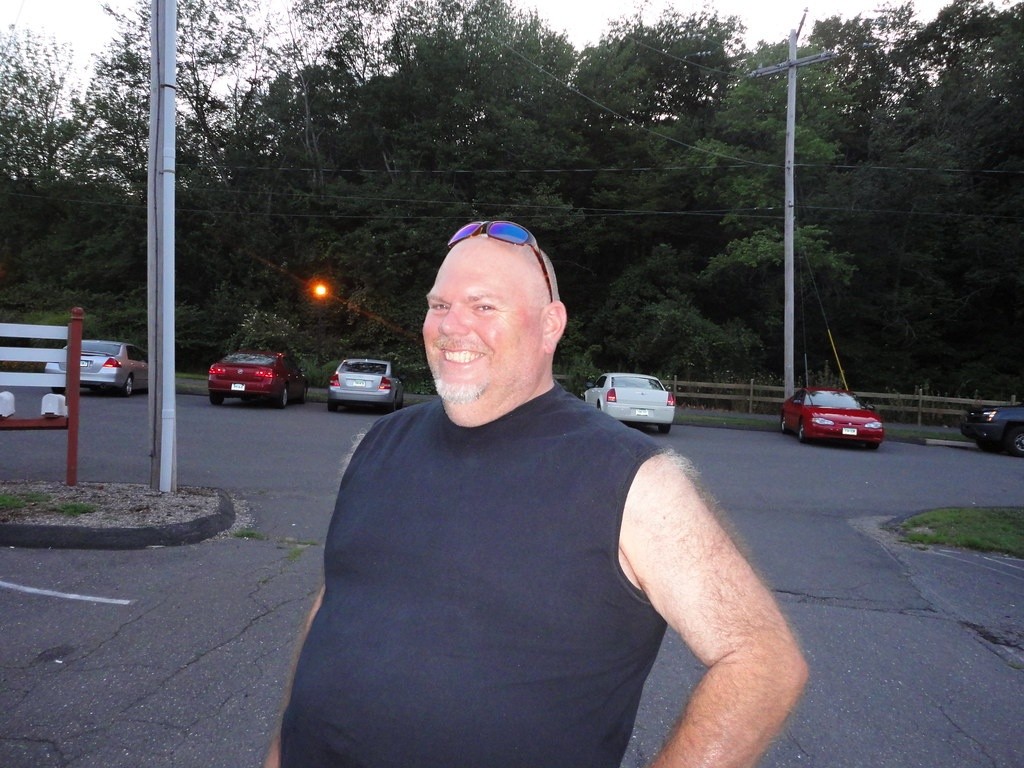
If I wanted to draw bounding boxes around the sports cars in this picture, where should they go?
[959,403,1024,457]
[780,387,884,451]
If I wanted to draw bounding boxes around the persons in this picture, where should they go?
[264,219,810,768]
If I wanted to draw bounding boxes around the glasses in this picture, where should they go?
[447,220,553,304]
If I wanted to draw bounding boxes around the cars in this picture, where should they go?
[327,359,405,414]
[583,373,675,435]
[207,347,309,409]
[45,340,148,397]
